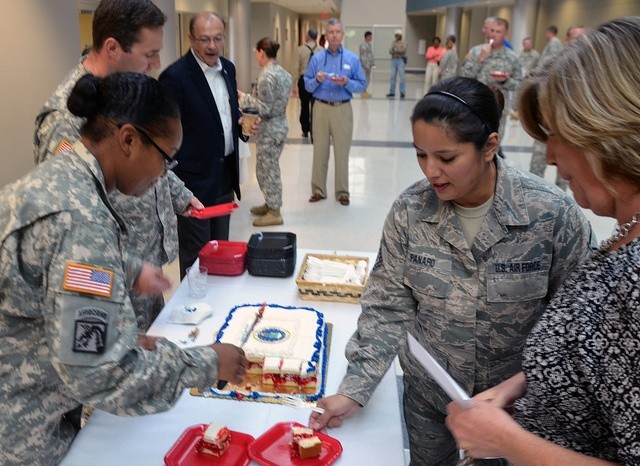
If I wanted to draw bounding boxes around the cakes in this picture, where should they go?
[289,426,323,459]
[194,423,234,458]
[205,302,327,403]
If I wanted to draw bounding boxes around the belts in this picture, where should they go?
[317,98,350,106]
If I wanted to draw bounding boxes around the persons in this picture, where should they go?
[385,30,410,99]
[158,12,263,285]
[310,78,599,466]
[302,18,368,205]
[481,16,515,50]
[518,36,538,76]
[540,26,564,61]
[461,18,520,160]
[424,35,445,95]
[438,33,459,82]
[237,36,292,227]
[443,16,640,466]
[360,31,376,101]
[34,1,165,165]
[0,71,251,465]
[293,28,321,144]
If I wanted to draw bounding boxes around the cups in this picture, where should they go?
[185,266,208,299]
[242,107,260,137]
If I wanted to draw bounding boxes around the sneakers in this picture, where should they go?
[252,209,283,225]
[251,202,269,214]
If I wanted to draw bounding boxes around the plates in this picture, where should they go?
[198,239,247,276]
[491,73,507,77]
[248,232,296,278]
[164,425,254,466]
[248,420,342,465]
[191,202,239,219]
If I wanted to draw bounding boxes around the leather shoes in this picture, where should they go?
[340,196,349,204]
[310,193,321,201]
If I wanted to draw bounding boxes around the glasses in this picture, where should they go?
[118,122,178,169]
[193,35,225,44]
